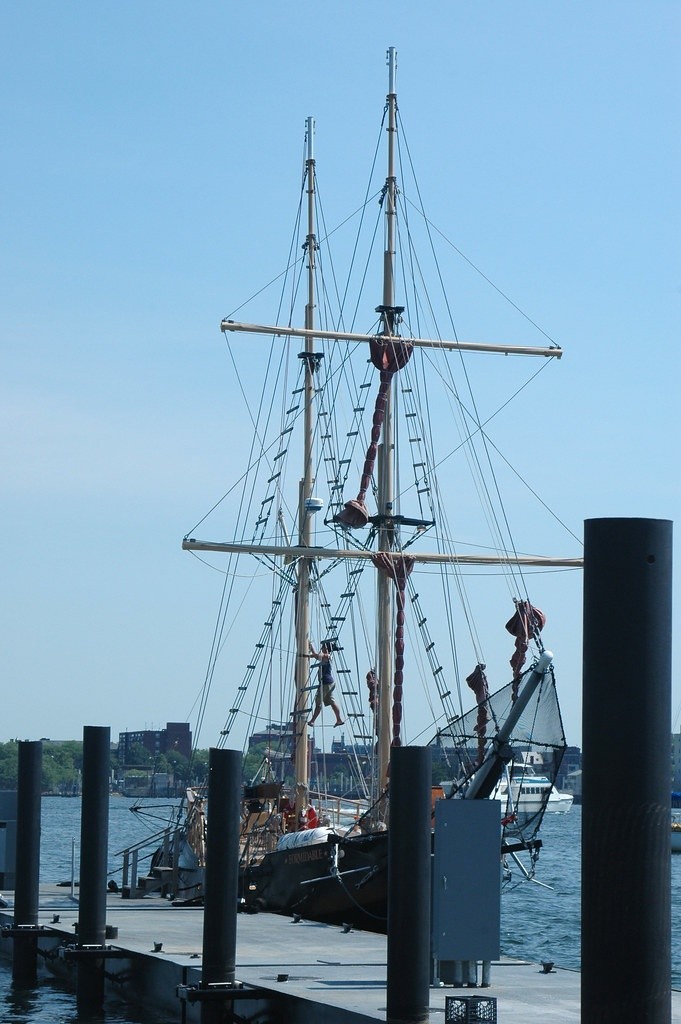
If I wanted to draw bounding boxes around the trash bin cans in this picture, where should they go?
[445,994,497,1024]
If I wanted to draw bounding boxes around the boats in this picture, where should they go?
[439,758,574,814]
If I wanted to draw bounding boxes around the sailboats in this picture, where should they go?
[114,48,584,930]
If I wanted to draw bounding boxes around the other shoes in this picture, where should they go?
[307,722,312,727]
[333,722,344,729]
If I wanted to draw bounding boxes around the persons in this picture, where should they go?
[307,639,344,728]
